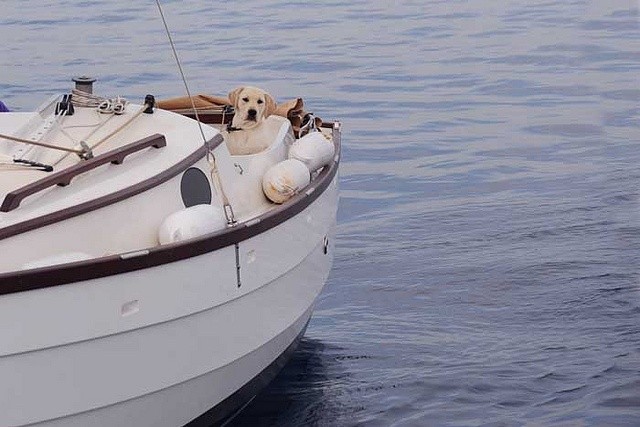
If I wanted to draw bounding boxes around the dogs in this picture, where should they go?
[220,85,282,155]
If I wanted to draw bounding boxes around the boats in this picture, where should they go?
[1,96,342,427]
